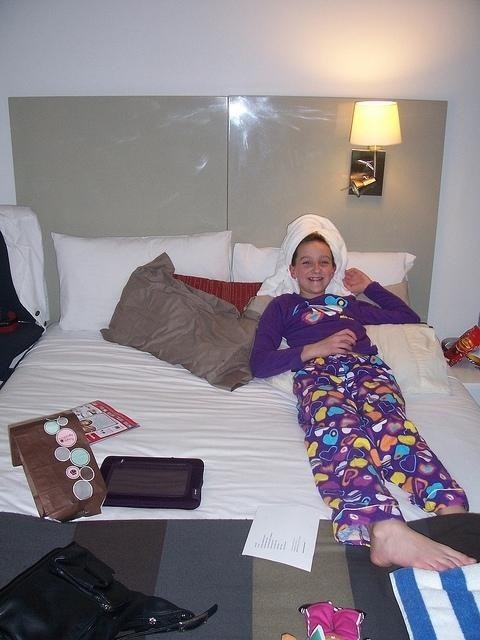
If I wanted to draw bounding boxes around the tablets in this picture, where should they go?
[95,454,205,510]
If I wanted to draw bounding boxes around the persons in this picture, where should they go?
[248,213,480,574]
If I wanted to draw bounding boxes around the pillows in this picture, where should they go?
[51,231,232,330]
[265,323,448,394]
[103,253,263,392]
[175,274,263,317]
[234,245,415,328]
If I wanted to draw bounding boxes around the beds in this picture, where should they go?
[0,326,479,640]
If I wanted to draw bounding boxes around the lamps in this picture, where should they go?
[346,102,401,197]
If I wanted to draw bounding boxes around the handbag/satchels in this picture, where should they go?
[0,541,220,640]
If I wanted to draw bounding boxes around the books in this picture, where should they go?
[70,400,139,445]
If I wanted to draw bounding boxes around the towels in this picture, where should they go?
[388,560,479,640]
